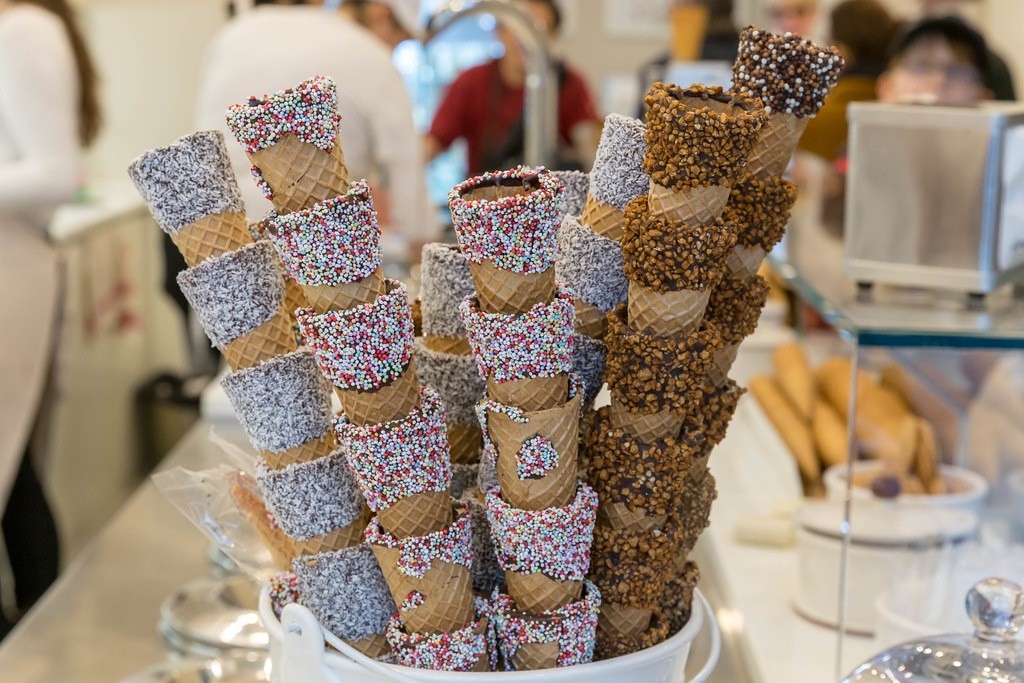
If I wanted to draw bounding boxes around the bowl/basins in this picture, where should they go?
[155,577,273,683]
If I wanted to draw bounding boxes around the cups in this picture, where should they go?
[795,460,985,638]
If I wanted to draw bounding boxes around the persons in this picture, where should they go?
[194,0,601,307]
[639,0,1022,346]
[0,1,102,632]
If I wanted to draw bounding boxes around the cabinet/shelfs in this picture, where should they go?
[0,97,1024,683]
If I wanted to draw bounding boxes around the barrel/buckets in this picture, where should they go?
[260,579,721,683]
